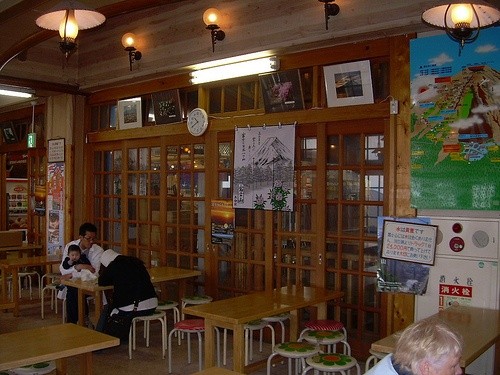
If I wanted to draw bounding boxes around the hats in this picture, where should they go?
[100,248,122,267]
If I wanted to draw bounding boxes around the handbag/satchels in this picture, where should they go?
[105,305,135,330]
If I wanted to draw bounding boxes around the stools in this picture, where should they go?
[0,243,500,375]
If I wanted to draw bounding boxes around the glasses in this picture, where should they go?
[83,237,97,241]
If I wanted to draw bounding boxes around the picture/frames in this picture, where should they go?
[0,121,18,143]
[323,59,374,108]
[258,68,305,113]
[47,138,66,162]
[115,97,143,130]
[151,89,183,126]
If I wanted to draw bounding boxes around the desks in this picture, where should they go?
[0,323,120,375]
[183,284,344,372]
[0,255,62,317]
[60,267,201,328]
[0,244,43,258]
[369,307,500,375]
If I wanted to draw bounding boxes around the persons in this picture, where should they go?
[56,245,95,291]
[364,318,463,375]
[57,223,104,328]
[95,250,158,332]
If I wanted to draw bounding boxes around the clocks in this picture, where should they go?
[186,108,208,137]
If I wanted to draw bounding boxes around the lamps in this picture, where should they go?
[121,32,141,71]
[421,0,500,48]
[35,0,106,65]
[203,8,225,53]
[318,0,340,30]
[27,101,38,148]
[0,85,36,98]
[188,57,280,86]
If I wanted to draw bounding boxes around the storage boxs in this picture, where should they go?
[138,199,190,266]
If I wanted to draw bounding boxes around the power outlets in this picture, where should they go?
[389,100,399,116]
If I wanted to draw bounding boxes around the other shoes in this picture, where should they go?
[85,317,94,329]
